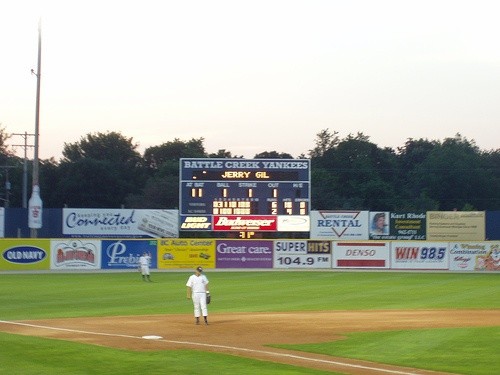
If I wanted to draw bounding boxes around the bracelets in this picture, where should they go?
[206,290,209,294]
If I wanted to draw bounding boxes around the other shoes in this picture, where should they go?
[205,321,208,325]
[196,321,199,325]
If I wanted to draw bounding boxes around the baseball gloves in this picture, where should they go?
[205,293,211,305]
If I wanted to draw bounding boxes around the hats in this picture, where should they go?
[196,266,203,272]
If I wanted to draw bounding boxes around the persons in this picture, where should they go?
[372,213,386,234]
[186,266,210,325]
[139,252,153,282]
